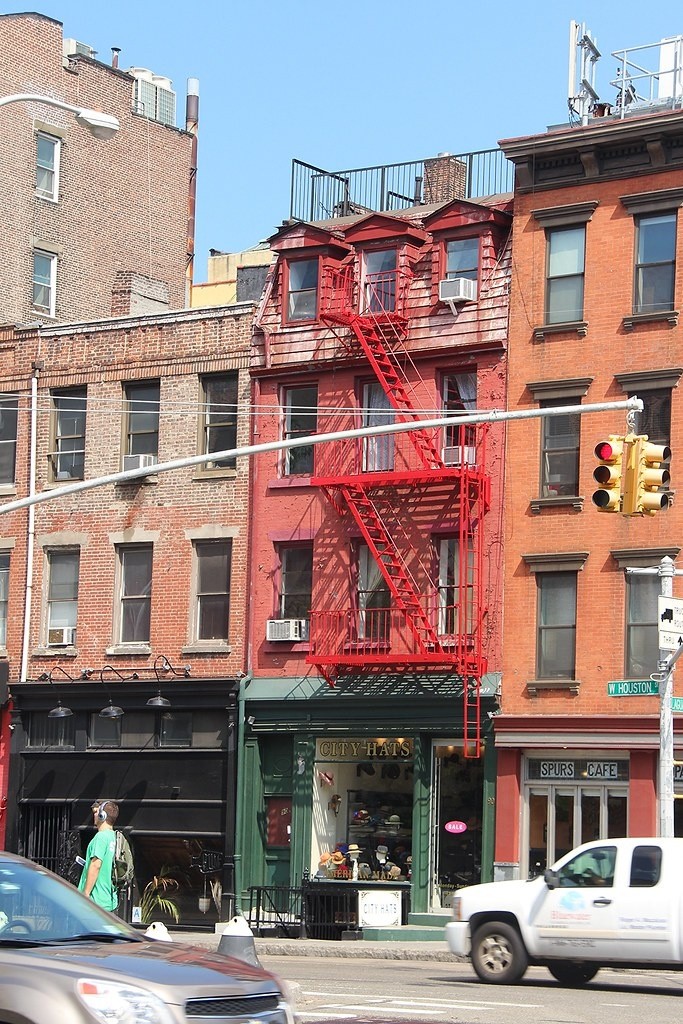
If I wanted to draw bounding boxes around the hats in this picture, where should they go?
[381,861,397,871]
[376,845,388,854]
[385,865,401,876]
[377,804,390,816]
[384,815,404,826]
[353,809,369,823]
[318,770,336,786]
[358,862,372,874]
[404,855,413,865]
[345,844,363,854]
[319,852,332,865]
[331,852,347,865]
[332,794,342,804]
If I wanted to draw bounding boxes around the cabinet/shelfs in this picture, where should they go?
[345,788,413,877]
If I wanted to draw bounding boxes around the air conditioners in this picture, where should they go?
[468,280,478,300]
[71,627,76,646]
[438,278,467,304]
[146,454,158,474]
[467,446,476,464]
[266,619,302,642]
[441,446,468,465]
[47,627,71,645]
[300,620,310,641]
[122,454,149,474]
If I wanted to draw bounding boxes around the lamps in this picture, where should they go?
[146,654,191,708]
[47,664,91,718]
[99,664,138,719]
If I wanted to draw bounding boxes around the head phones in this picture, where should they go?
[97,801,109,822]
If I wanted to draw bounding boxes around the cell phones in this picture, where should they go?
[76,856,87,866]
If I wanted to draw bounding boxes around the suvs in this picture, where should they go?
[0,850,295,1024]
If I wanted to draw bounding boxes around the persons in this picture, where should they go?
[585,852,615,884]
[78,802,119,913]
[374,845,390,863]
[346,844,363,861]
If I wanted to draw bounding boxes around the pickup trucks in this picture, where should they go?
[444,837,682,984]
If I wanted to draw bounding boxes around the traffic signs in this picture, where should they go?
[657,595,683,651]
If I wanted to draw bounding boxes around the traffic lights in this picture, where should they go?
[622,433,671,518]
[591,435,623,514]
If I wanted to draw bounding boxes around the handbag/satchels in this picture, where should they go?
[112,829,137,900]
